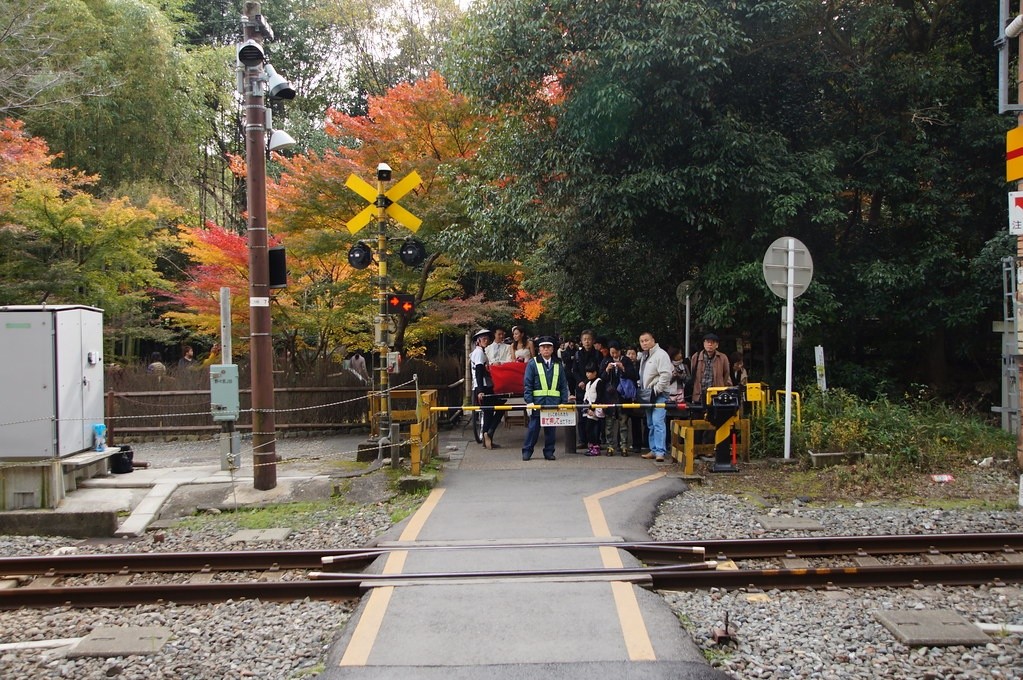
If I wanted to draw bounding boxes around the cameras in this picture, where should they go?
[610,363,616,367]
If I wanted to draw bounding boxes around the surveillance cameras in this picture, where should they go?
[264,64,296,101]
[236,39,264,68]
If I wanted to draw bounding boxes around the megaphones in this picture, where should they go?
[377,163,392,181]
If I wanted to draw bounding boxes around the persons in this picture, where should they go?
[521,336,568,461]
[469,329,504,449]
[179,345,197,368]
[510,326,536,362]
[148,351,166,381]
[533,324,748,456]
[484,328,511,364]
[637,332,672,461]
[349,348,369,380]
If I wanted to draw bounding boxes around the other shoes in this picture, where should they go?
[584,444,601,456]
[544,454,556,460]
[621,448,629,456]
[522,454,531,461]
[642,451,655,459]
[656,454,665,461]
[481,432,501,449]
[606,448,614,455]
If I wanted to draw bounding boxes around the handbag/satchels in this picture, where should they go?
[684,381,694,402]
[616,377,637,399]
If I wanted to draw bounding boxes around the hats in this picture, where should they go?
[471,328,493,339]
[704,334,720,341]
[537,336,555,345]
